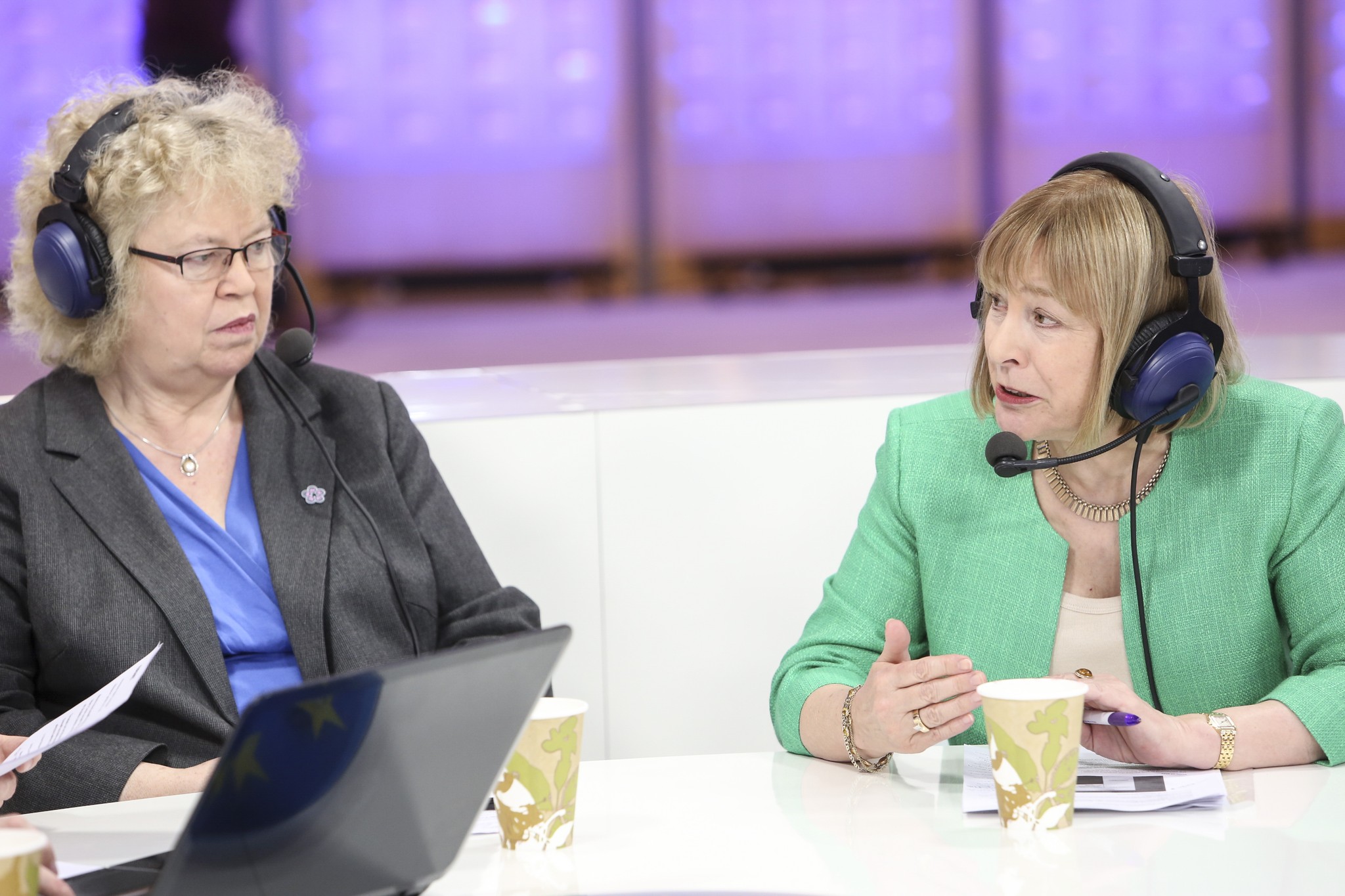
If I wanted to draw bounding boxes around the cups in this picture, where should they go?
[0,828,48,896]
[493,697,589,853]
[976,677,1089,831]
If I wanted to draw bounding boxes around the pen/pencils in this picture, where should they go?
[1081,707,1142,726]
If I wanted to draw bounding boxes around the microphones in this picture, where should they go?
[274,258,318,369]
[986,384,1192,470]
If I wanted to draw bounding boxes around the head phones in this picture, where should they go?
[968,154,1219,427]
[30,95,290,319]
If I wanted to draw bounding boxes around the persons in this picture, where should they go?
[0,732,79,895]
[767,152,1345,775]
[0,60,553,822]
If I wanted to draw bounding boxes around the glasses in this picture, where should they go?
[128,228,292,281]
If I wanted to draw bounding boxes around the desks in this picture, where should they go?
[0,744,1345,895]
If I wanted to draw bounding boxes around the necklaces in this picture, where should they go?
[99,382,235,477]
[1038,438,1172,524]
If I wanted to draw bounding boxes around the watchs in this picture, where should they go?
[1200,711,1237,771]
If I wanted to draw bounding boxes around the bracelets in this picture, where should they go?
[842,684,893,773]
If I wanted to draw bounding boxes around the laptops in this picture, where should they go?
[40,622,571,896]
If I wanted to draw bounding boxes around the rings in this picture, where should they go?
[910,709,932,732]
[1075,668,1092,679]
